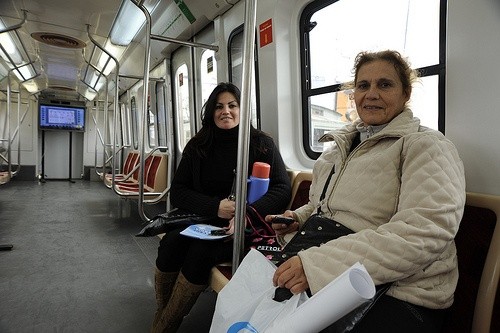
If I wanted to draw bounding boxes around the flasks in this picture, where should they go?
[240,162,270,202]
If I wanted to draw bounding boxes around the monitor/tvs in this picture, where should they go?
[39,104,85,130]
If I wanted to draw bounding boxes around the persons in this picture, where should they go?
[265,51,465,332]
[150,83,292,333]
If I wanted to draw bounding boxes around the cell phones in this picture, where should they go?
[272,216,293,224]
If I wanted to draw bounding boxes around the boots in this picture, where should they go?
[152,269,209,333]
[153,264,179,333]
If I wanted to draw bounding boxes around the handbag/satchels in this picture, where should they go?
[134,207,217,237]
[209,249,310,333]
[275,214,395,333]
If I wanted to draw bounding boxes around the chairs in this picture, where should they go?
[101,150,168,222]
[210,169,500,333]
